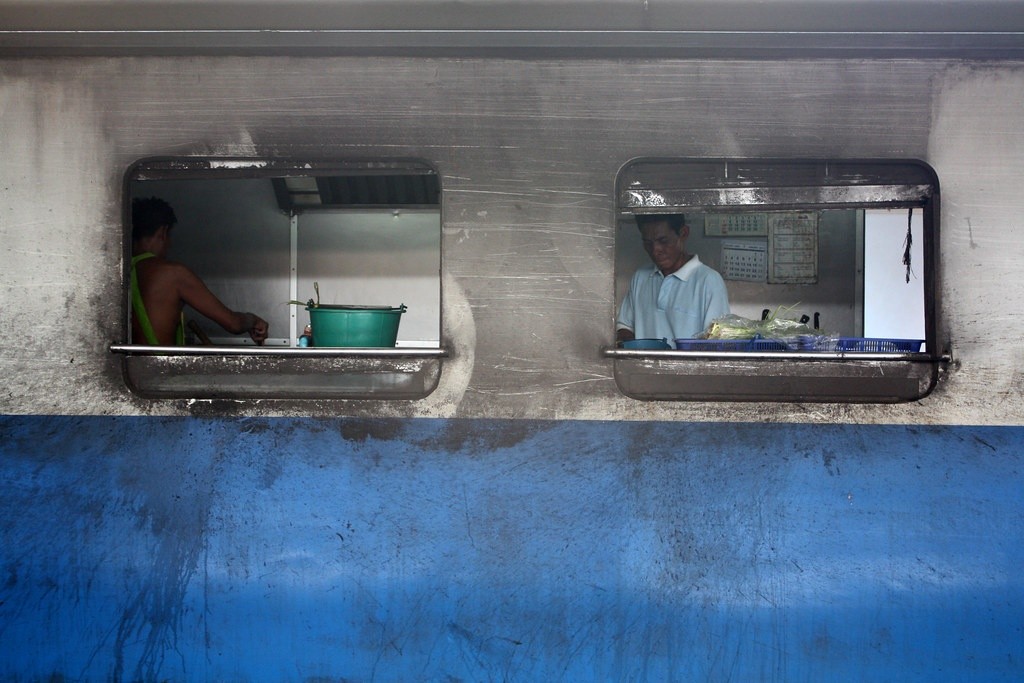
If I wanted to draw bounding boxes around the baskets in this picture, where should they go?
[796,333,926,353]
[622,337,672,351]
[675,339,790,353]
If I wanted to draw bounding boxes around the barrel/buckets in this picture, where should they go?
[305,298,408,347]
[617,337,671,349]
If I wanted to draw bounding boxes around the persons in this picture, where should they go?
[129,198,268,348]
[615,213,729,344]
